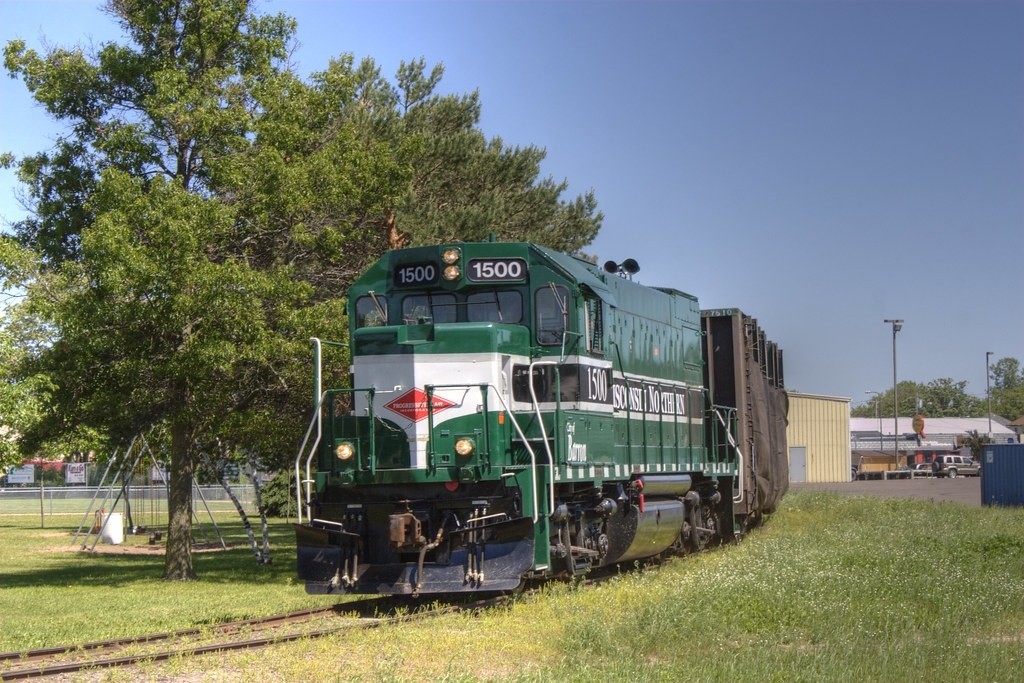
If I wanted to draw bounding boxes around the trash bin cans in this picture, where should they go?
[100,512,124,545]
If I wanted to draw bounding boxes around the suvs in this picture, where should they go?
[934,455,981,479]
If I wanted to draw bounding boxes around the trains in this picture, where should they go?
[296,241,792,593]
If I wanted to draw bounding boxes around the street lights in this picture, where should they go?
[986,351,996,443]
[865,390,884,452]
[884,319,905,471]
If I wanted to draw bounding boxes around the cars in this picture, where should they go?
[908,463,933,477]
